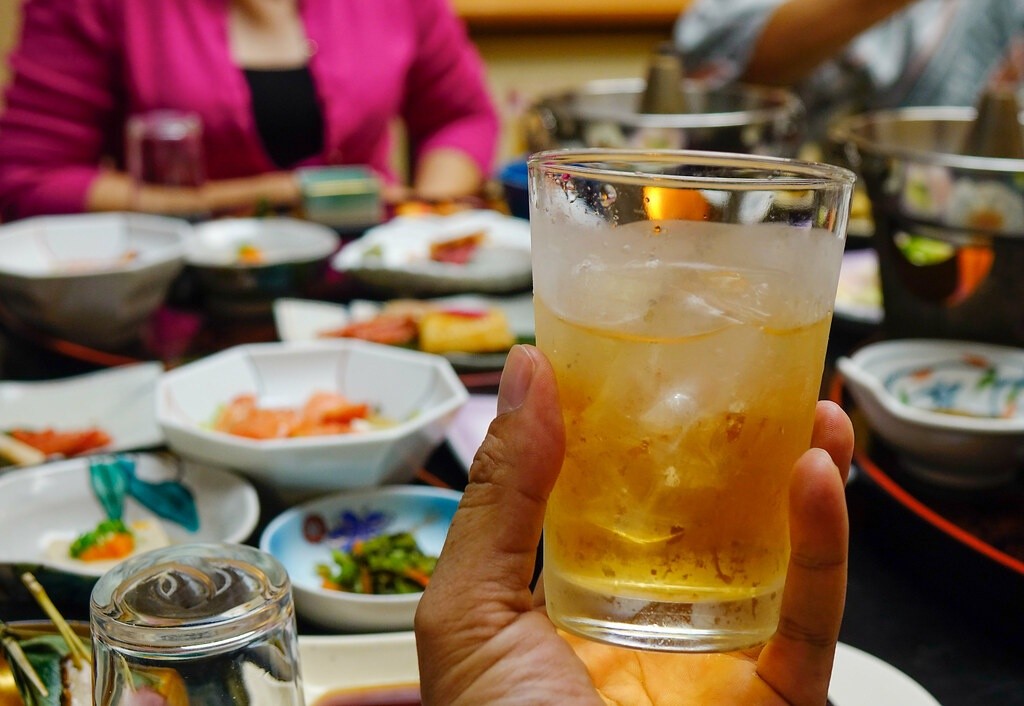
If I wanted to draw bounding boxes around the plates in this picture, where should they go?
[1,450,466,705]
[331,209,533,296]
[272,295,537,371]
[0,361,166,472]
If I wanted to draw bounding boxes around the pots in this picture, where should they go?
[827,81,1024,238]
[531,46,804,158]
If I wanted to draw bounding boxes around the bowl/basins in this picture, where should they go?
[153,337,470,508]
[835,339,1023,488]
[2,211,342,346]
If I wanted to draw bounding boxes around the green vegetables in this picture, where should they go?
[315,531,439,596]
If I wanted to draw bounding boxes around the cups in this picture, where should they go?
[90,542,306,706]
[124,109,204,222]
[525,149,857,653]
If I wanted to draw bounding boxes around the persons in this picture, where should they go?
[676,0,1024,186]
[411,335,853,706]
[0,0,501,368]
